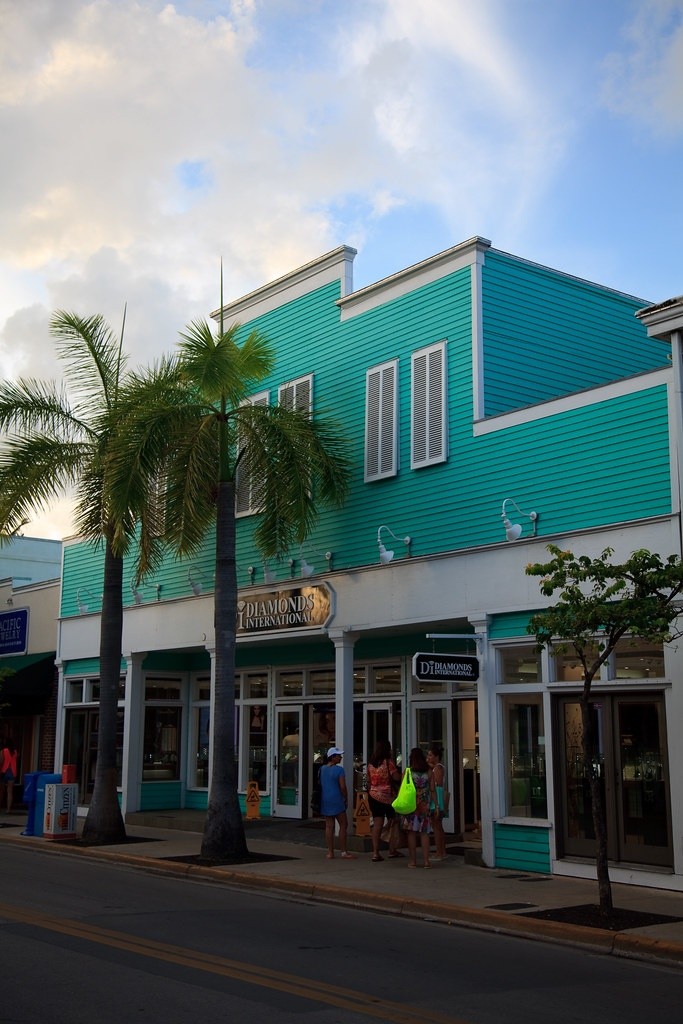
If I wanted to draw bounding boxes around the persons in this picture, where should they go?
[423,745,450,862]
[313,710,337,744]
[367,736,407,862]
[620,733,634,770]
[317,747,354,858]
[1,738,21,815]
[251,705,268,733]
[400,747,441,871]
[283,726,301,746]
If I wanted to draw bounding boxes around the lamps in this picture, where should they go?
[76,586,104,616]
[187,565,215,596]
[377,525,411,565]
[501,498,538,542]
[261,552,294,585]
[299,540,332,579]
[130,576,160,605]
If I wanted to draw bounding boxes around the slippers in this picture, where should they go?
[424,861,432,868]
[428,854,449,861]
[408,864,416,868]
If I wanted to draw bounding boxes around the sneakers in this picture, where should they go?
[341,852,353,858]
[325,853,334,858]
[326,747,345,758]
[388,849,405,857]
[372,854,384,861]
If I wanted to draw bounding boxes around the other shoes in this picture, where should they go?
[6,810,12,815]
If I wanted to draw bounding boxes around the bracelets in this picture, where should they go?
[435,805,439,810]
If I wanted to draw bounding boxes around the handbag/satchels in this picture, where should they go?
[311,781,322,812]
[391,767,416,815]
[429,785,445,812]
